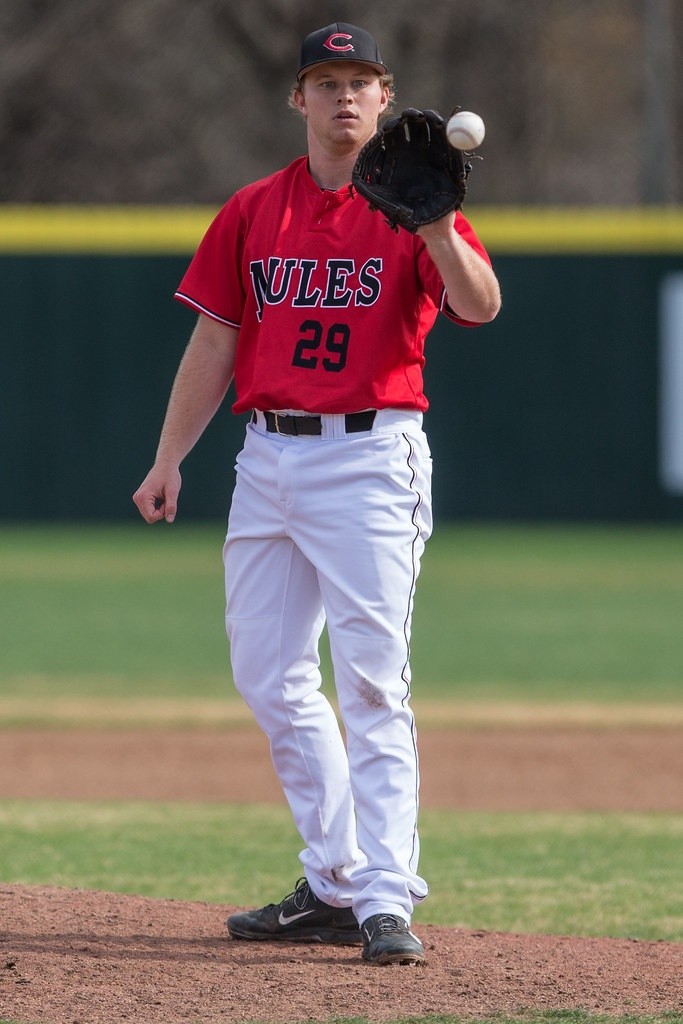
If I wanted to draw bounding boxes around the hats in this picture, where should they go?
[296,23,388,85]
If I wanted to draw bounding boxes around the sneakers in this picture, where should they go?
[360,914,425,967]
[226,877,364,946]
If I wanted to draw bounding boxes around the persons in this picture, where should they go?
[132,21,502,967]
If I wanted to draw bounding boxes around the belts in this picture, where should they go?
[251,409,377,437]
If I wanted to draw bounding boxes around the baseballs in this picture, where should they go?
[446,111,486,150]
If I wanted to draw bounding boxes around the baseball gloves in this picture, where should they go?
[352,107,467,234]
